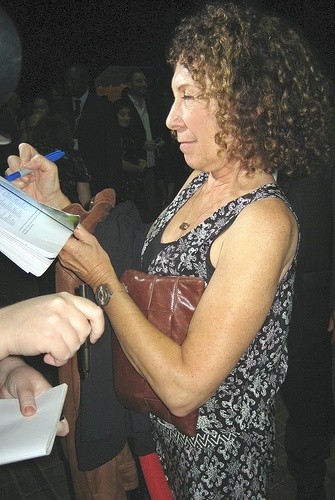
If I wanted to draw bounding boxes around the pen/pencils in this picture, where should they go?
[3,148,67,183]
[79,284,92,384]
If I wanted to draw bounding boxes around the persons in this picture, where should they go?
[0,288,106,439]
[2,2,331,500]
[0,59,194,226]
[273,166,334,500]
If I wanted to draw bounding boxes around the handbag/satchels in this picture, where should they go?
[112,268,206,439]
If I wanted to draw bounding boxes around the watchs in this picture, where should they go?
[94,282,129,305]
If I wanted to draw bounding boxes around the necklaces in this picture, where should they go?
[179,173,259,230]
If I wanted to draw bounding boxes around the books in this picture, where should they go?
[0,176,82,278]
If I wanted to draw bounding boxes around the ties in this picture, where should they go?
[75,100,81,118]
